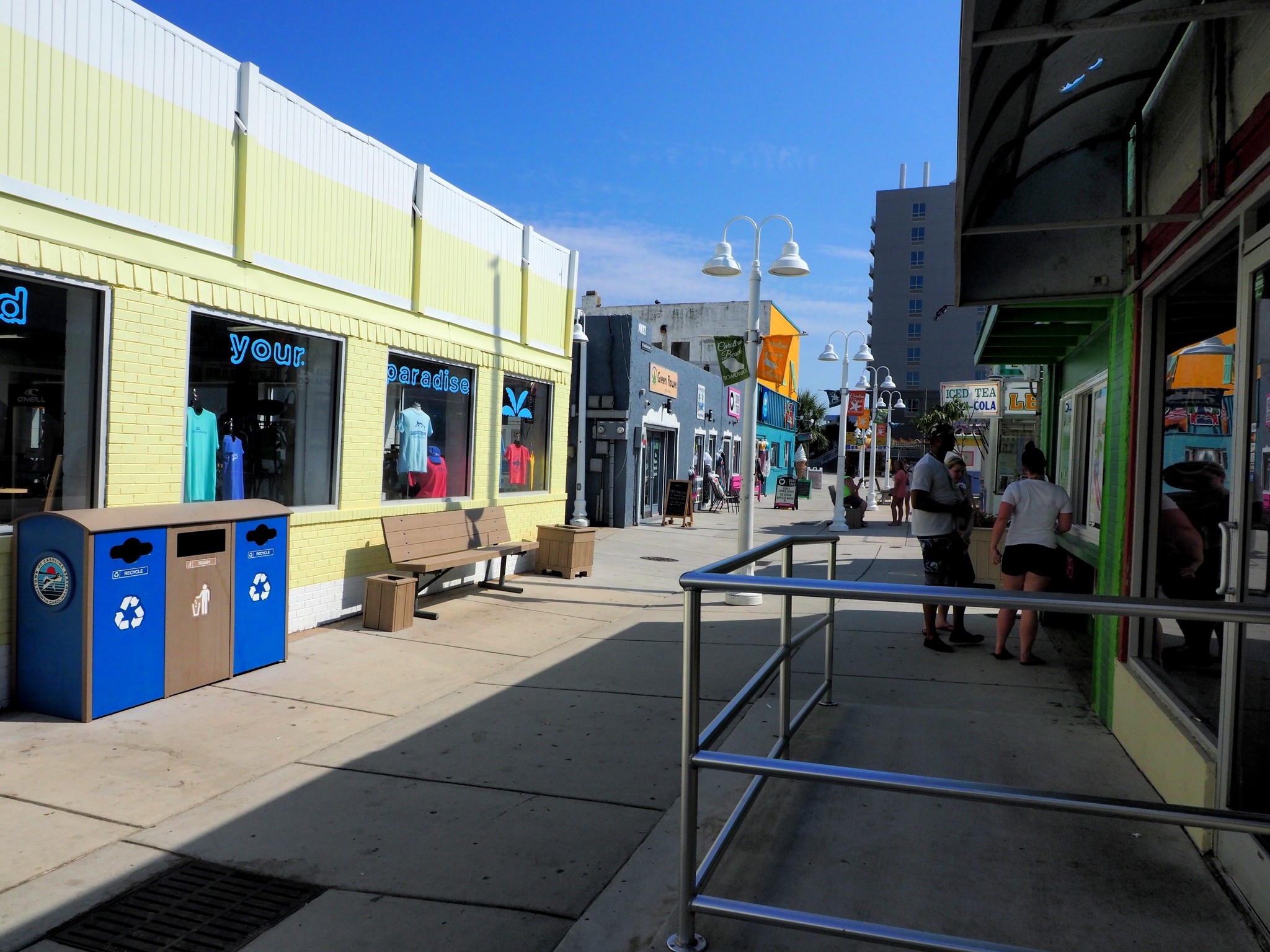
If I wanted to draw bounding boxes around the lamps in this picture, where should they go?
[733,417,739,425]
[784,413,803,420]
[794,418,814,423]
[933,302,954,320]
[662,399,672,414]
[706,410,713,421]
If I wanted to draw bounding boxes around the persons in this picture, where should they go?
[1166,457,1230,664]
[765,440,769,462]
[704,449,713,503]
[688,465,697,500]
[843,464,868,527]
[887,459,906,526]
[892,455,912,522]
[719,446,726,490]
[1154,491,1204,662]
[910,422,986,652]
[759,439,766,472]
[716,449,723,484]
[989,442,1073,663]
[397,403,434,473]
[922,451,976,636]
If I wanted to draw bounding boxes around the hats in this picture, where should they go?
[427,446,442,463]
[927,421,953,443]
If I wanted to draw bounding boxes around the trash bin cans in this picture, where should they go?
[10,497,294,723]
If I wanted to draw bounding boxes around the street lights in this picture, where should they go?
[568,308,592,527]
[818,331,874,536]
[877,391,909,496]
[855,365,897,513]
[699,216,814,607]
[852,417,873,489]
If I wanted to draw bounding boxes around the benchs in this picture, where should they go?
[875,478,892,506]
[829,485,861,529]
[730,475,761,502]
[380,505,539,621]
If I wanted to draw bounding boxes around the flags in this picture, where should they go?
[847,390,866,416]
[713,335,749,387]
[888,422,901,428]
[857,409,870,429]
[864,438,871,450]
[856,438,863,449]
[756,334,793,385]
[873,409,890,425]
[824,390,841,408]
[877,423,886,437]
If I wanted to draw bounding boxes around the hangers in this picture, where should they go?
[514,435,522,447]
[227,420,236,440]
[189,390,203,413]
[531,448,533,457]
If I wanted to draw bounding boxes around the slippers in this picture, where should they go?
[1020,654,1046,666]
[996,649,1016,660]
[922,629,927,636]
[936,625,953,632]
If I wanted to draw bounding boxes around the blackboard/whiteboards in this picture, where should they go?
[663,479,693,519]
[797,479,813,498]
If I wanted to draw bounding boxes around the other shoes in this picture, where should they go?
[924,634,954,652]
[948,630,985,643]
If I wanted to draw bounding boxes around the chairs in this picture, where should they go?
[707,477,741,515]
